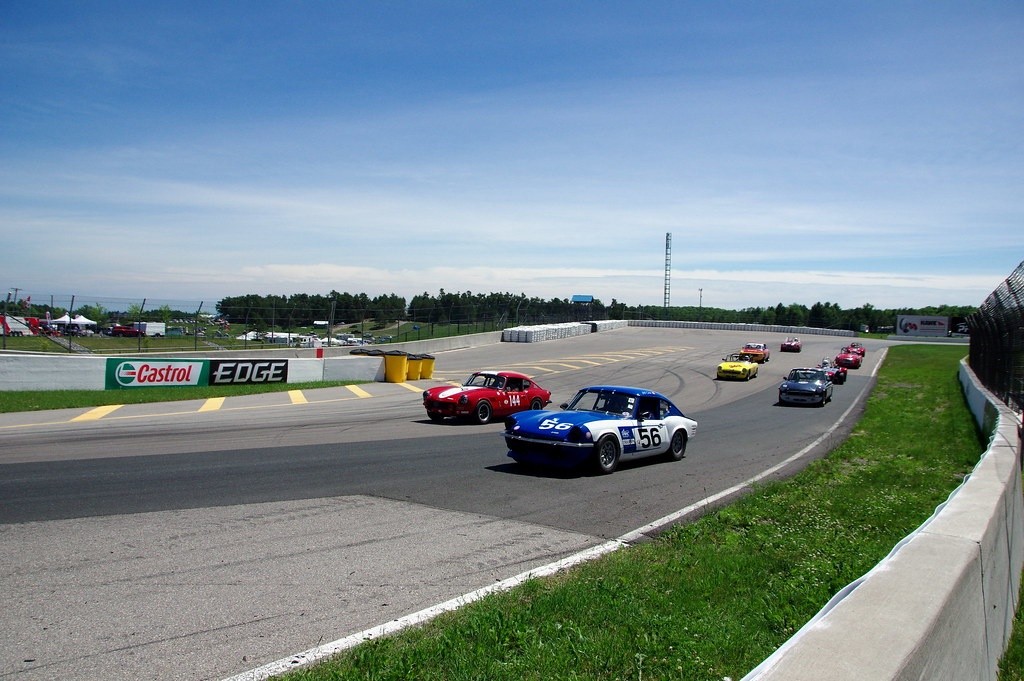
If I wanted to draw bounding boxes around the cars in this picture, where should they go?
[779,366,835,406]
[717,352,762,381]
[847,340,866,355]
[815,357,848,382]
[502,385,698,475]
[779,336,803,351]
[40,318,393,347]
[836,347,863,369]
[742,343,771,362]
[421,369,555,426]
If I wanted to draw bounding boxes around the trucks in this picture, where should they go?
[0,314,40,336]
